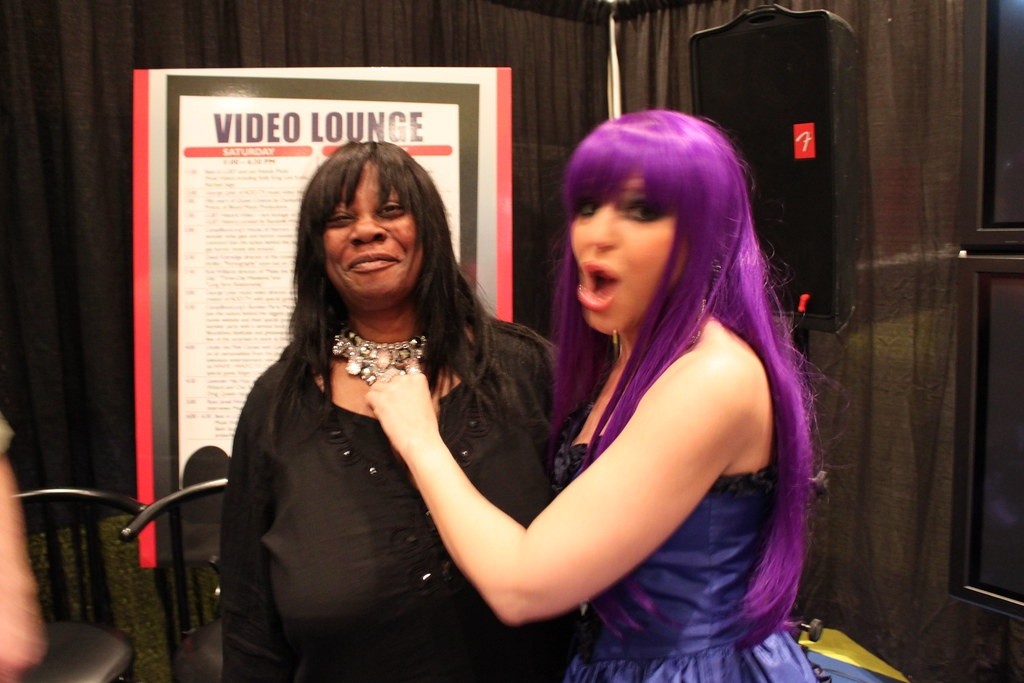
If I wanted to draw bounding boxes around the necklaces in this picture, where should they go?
[334,331,430,389]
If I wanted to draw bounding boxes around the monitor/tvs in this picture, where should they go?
[940,0,1024,625]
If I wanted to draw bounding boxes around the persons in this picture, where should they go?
[218,140,580,682]
[367,109,820,683]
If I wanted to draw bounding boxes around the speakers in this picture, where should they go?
[688,4,864,334]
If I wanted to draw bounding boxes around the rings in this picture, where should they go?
[379,368,401,381]
[405,364,422,374]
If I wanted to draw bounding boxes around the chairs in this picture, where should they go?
[11,486,154,683]
[121,474,226,682]
